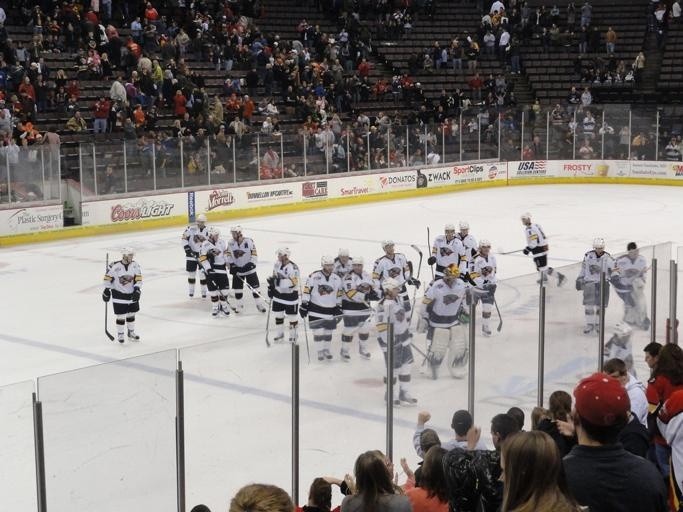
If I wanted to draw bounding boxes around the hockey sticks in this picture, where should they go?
[194,255,239,315]
[409,244,422,329]
[410,343,436,382]
[493,299,502,332]
[493,249,525,255]
[265,299,271,347]
[299,279,310,365]
[236,273,270,305]
[427,227,434,280]
[104,253,114,341]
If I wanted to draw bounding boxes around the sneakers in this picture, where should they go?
[212,309,219,316]
[584,325,592,333]
[556,275,564,286]
[359,350,371,357]
[202,292,206,298]
[118,332,124,342]
[258,303,266,312]
[235,304,243,313]
[288,334,296,342]
[221,307,230,315]
[399,392,417,403]
[128,331,139,339]
[189,292,194,297]
[274,333,283,340]
[537,278,547,282]
[323,350,332,359]
[318,352,323,360]
[340,351,350,359]
[384,392,400,405]
[482,327,491,335]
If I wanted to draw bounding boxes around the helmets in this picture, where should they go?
[520,212,531,218]
[593,238,605,249]
[382,240,393,249]
[443,263,459,275]
[277,247,290,256]
[198,214,207,221]
[208,226,219,235]
[351,257,362,264]
[321,256,334,266]
[614,320,632,338]
[445,223,454,230]
[231,225,241,234]
[479,240,491,248]
[338,247,348,256]
[460,222,470,229]
[121,248,135,255]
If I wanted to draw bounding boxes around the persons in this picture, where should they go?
[615,242,651,331]
[1,0,683,207]
[575,235,621,335]
[521,215,568,287]
[101,247,143,344]
[181,212,497,408]
[189,342,683,512]
[603,320,638,379]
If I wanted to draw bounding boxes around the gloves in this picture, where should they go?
[367,293,379,299]
[230,264,237,274]
[268,287,273,297]
[482,266,492,276]
[408,277,419,285]
[428,257,436,264]
[299,305,307,318]
[523,246,531,255]
[388,267,400,277]
[184,245,192,255]
[133,287,140,300]
[576,278,583,290]
[205,269,215,280]
[103,290,110,301]
[335,307,342,322]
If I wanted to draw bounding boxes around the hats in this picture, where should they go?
[452,410,472,425]
[573,372,631,426]
[382,279,400,291]
